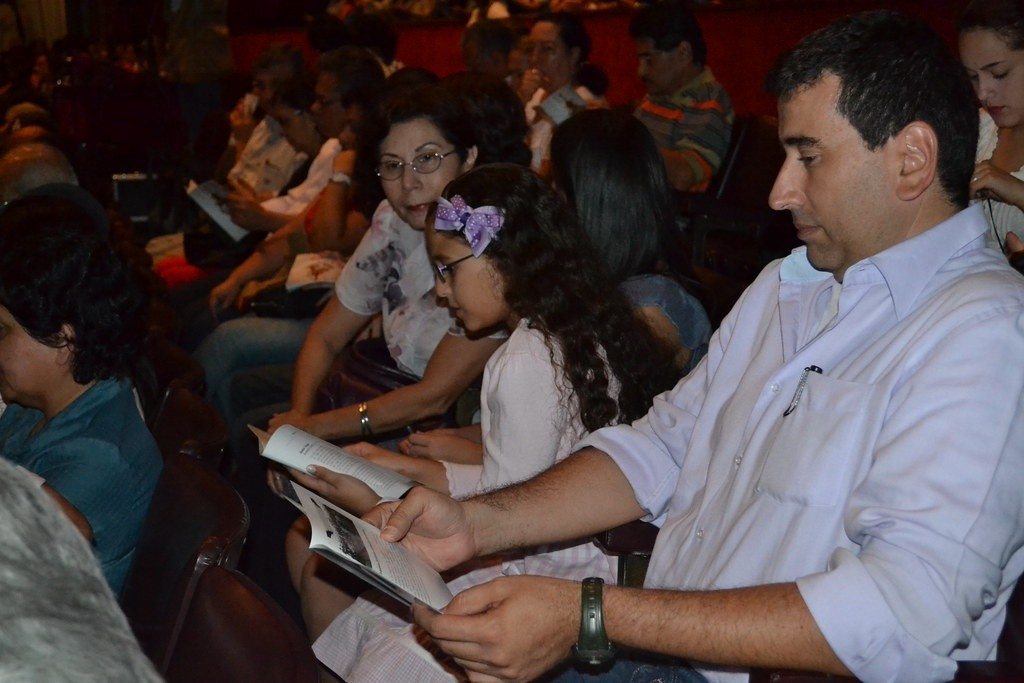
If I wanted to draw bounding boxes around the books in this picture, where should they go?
[282,481,460,624]
[247,418,422,506]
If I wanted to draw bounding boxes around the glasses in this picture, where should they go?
[275,111,306,126]
[432,252,492,283]
[365,148,463,180]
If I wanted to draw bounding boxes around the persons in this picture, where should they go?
[1,185,166,622]
[949,2,1024,272]
[195,113,392,495]
[4,0,742,316]
[540,107,715,381]
[248,163,680,682]
[270,15,1022,681]
[241,89,515,509]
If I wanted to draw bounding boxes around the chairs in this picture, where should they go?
[119,454,249,676]
[148,381,228,469]
[165,566,320,683]
[135,326,206,429]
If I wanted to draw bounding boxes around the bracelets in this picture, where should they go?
[357,402,375,436]
